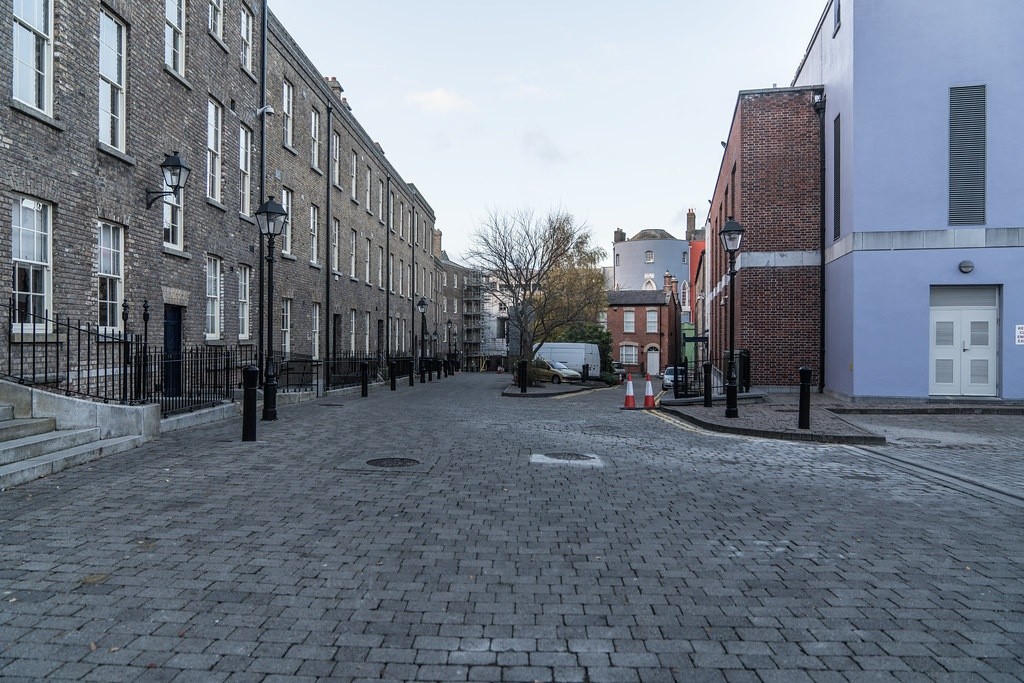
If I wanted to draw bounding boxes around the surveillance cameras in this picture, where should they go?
[334,275,338,281]
[265,107,274,116]
[720,298,725,306]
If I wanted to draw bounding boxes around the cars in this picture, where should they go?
[527,359,582,386]
[664,366,688,390]
[658,370,667,379]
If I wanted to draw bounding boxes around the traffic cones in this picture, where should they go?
[640,373,659,410]
[620,372,643,410]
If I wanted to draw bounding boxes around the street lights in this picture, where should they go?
[446,319,453,373]
[254,193,290,420]
[417,295,427,378]
[718,214,744,418]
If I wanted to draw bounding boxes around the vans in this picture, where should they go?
[531,341,601,380]
[611,362,627,382]
